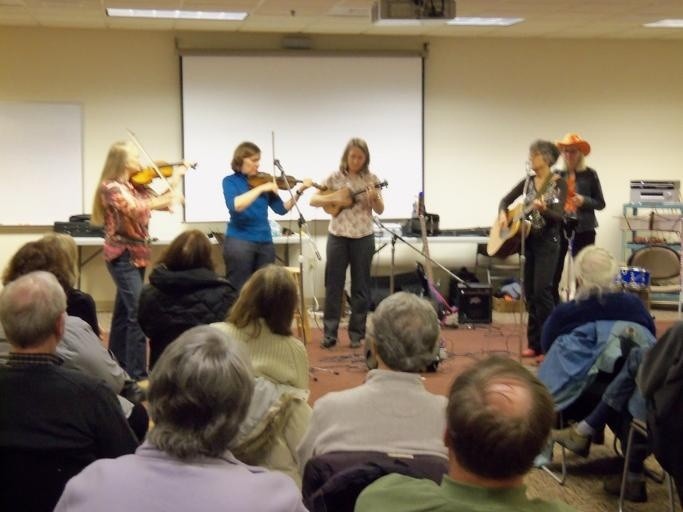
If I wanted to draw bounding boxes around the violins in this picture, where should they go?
[130,160,197,186]
[246,171,326,191]
[322,179,387,217]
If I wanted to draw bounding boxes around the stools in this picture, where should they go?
[281,266,313,345]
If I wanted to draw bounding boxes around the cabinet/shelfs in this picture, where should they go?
[617,204,683,255]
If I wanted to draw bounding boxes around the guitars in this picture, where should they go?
[415,191,454,320]
[486,187,561,259]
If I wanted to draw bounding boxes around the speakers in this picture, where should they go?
[457,284,491,325]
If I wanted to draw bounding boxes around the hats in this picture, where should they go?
[556,133,590,156]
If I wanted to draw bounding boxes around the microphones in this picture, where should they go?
[372,216,382,228]
[525,159,532,173]
[273,160,284,175]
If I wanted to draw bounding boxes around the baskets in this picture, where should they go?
[492,295,527,313]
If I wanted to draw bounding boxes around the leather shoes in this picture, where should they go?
[349,340,360,348]
[535,353,544,362]
[522,349,536,357]
[321,338,337,347]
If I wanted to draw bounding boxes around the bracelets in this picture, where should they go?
[295,187,305,197]
[370,193,382,202]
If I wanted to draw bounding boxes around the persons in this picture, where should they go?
[224,144,314,296]
[93,139,191,383]
[293,291,566,511]
[554,133,604,299]
[498,142,569,364]
[55,230,313,510]
[2,234,149,511]
[542,245,683,503]
[309,136,386,349]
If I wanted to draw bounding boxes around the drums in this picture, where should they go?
[618,265,651,290]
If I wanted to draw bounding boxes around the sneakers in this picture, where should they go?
[552,424,591,458]
[605,477,647,503]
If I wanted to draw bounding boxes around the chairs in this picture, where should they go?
[235,376,313,489]
[624,244,683,318]
[533,324,660,488]
[298,445,449,512]
[609,399,682,512]
[471,239,523,287]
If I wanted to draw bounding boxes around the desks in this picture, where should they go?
[65,233,219,297]
[257,231,308,270]
[366,231,521,315]
